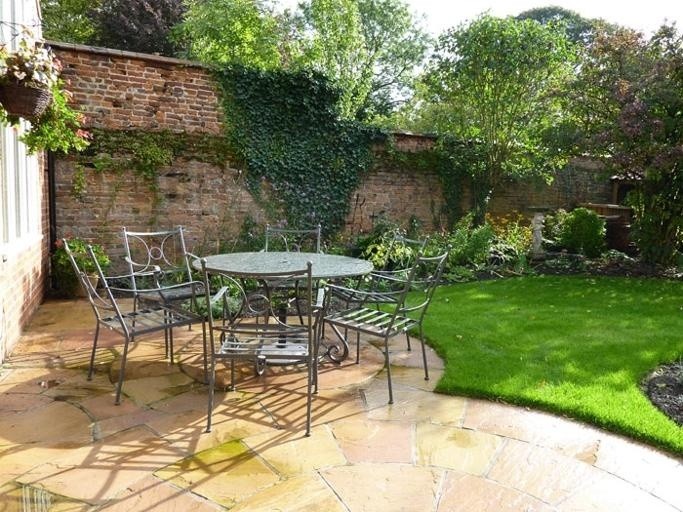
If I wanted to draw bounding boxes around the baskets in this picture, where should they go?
[0,84,53,119]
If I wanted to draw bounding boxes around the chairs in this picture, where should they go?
[316,230,430,363]
[53,236,209,405]
[194,251,323,441]
[310,248,451,411]
[118,215,233,383]
[244,223,324,344]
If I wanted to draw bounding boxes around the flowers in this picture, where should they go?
[0,26,90,153]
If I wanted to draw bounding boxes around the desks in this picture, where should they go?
[185,252,375,395]
